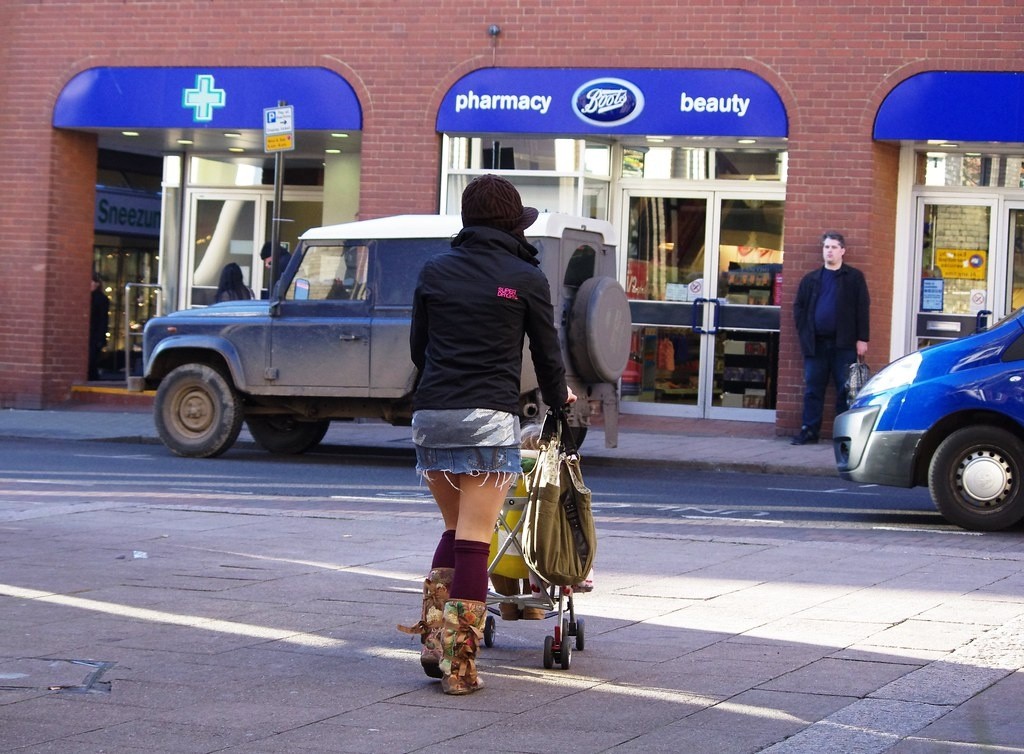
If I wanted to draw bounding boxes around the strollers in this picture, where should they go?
[484,396,595,671]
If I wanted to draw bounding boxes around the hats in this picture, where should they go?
[462,174,539,235]
[261,240,282,259]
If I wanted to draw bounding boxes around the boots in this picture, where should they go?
[438,598,484,695]
[397,568,446,678]
[490,572,521,620]
[520,579,545,620]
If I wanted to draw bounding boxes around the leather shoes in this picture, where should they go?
[791,425,821,444]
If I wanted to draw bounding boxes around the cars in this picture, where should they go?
[830,306,1024,530]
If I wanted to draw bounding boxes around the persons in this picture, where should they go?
[792,230,870,446]
[408,174,578,696]
[88,276,110,380]
[260,242,292,283]
[217,263,250,302]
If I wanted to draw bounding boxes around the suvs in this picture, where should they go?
[143,216,633,458]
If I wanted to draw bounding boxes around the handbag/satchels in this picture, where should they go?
[845,354,870,406]
[520,415,596,582]
